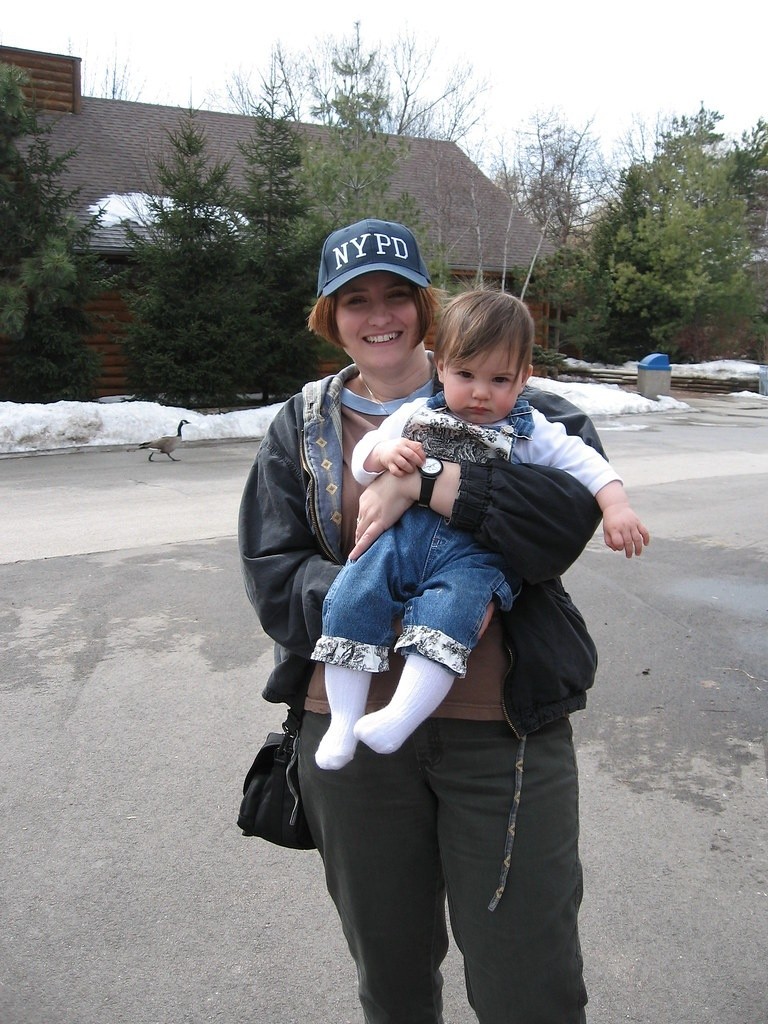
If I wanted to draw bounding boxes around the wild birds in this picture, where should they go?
[138,420,190,462]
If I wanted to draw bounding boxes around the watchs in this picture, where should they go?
[414,456,444,509]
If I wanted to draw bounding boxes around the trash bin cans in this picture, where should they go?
[636,352,673,402]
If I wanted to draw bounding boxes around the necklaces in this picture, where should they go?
[362,375,414,417]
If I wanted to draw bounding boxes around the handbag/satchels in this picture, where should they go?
[237,728,318,850]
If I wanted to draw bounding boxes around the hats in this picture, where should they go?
[315,219,431,297]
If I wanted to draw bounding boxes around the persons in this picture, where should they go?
[309,289,650,770]
[238,218,609,1024]
